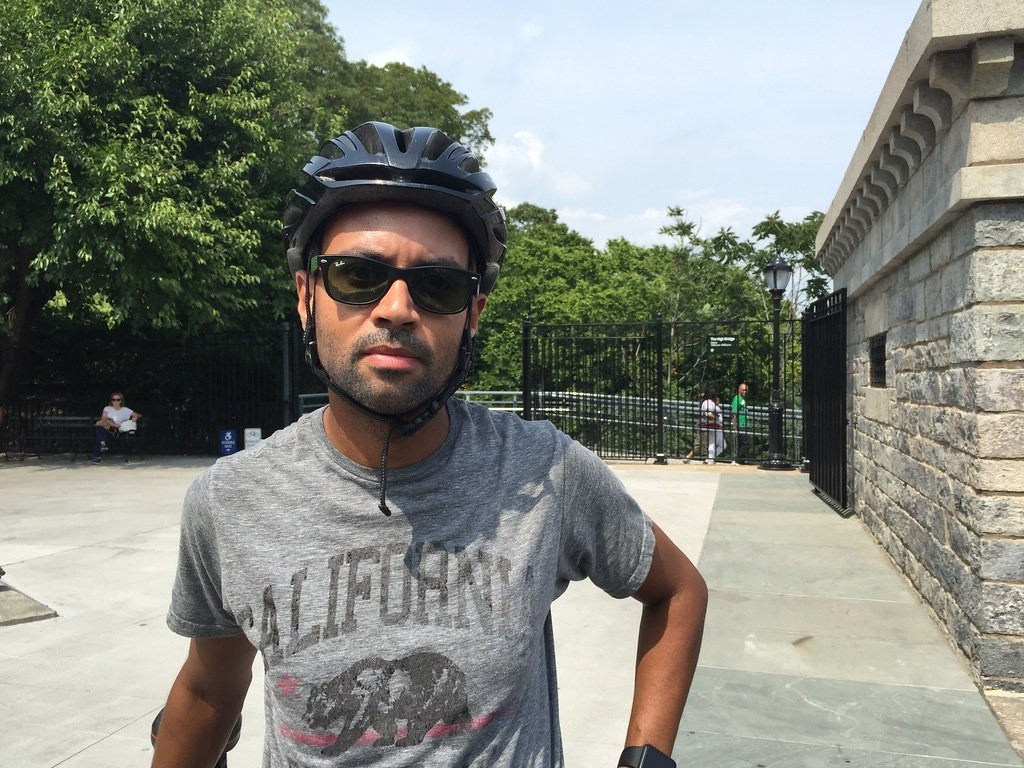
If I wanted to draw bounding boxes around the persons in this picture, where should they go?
[148,118,708,768]
[702,388,726,465]
[92,393,143,464]
[681,386,708,465]
[731,383,750,465]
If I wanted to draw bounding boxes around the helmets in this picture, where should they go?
[281,121,508,296]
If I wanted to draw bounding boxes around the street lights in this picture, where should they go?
[760,255,799,472]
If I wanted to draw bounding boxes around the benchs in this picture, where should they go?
[16,413,150,465]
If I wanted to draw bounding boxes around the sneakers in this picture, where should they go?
[93,457,102,464]
[100,444,108,452]
[683,456,741,466]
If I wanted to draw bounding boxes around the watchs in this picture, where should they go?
[616,743,677,767]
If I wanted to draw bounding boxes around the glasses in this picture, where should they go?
[111,399,122,401]
[308,254,481,315]
